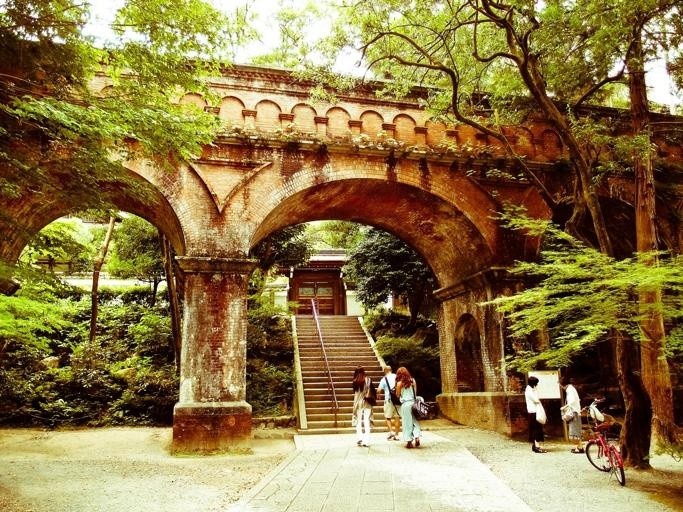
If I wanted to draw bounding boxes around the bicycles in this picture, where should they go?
[580,397,625,486]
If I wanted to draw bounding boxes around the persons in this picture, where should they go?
[377,366,401,440]
[560,376,585,454]
[352,366,372,448]
[524,376,547,453]
[396,366,423,449]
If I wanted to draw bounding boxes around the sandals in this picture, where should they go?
[532,445,547,453]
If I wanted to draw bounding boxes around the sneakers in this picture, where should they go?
[357,439,369,446]
[387,431,419,448]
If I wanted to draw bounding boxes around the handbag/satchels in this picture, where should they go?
[536,403,547,425]
[364,387,376,406]
[391,389,401,405]
[560,405,575,422]
[411,400,430,420]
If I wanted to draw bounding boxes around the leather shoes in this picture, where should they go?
[571,447,584,453]
